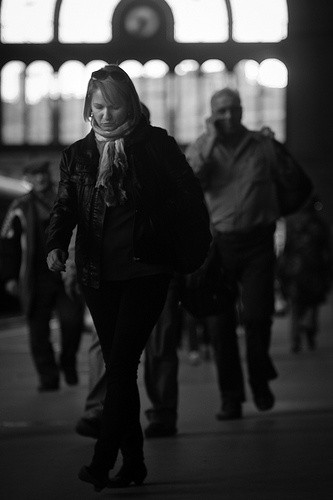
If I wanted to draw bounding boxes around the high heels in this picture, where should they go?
[108,465,148,488]
[78,465,111,493]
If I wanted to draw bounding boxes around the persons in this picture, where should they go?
[46,64,210,493]
[186,88,332,421]
[75,102,184,438]
[0,162,83,391]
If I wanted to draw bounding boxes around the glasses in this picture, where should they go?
[91,70,127,81]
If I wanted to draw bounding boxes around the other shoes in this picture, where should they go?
[251,388,275,412]
[58,361,78,387]
[144,423,172,439]
[37,382,60,392]
[215,410,243,420]
